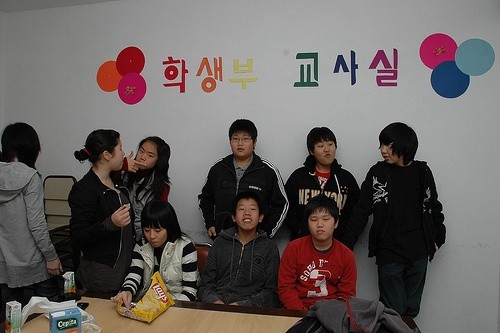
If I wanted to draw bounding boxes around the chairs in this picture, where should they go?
[43,175,78,243]
[194,243,212,275]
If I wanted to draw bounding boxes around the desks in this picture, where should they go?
[0,290,316,333]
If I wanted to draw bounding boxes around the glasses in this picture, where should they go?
[231,135,252,143]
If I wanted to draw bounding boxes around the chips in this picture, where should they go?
[136,297,160,313]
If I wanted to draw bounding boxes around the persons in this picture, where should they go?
[197,118,292,242]
[276,193,357,311]
[281,126,363,251]
[195,191,281,309]
[67,128,138,300]
[0,121,65,322]
[341,122,447,320]
[111,198,202,311]
[112,135,173,242]
